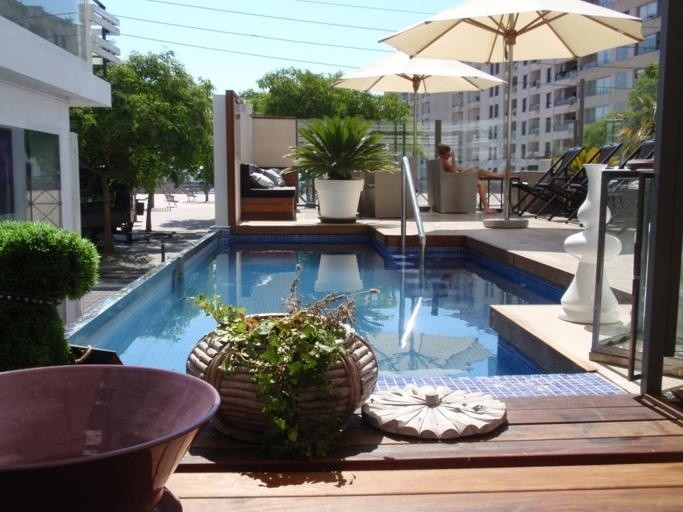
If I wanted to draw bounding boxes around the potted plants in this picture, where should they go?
[280,118,405,223]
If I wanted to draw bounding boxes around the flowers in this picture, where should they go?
[189,263,379,458]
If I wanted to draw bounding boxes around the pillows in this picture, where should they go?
[249,171,274,189]
[259,166,285,186]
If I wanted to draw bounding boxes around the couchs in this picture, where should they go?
[240,160,298,224]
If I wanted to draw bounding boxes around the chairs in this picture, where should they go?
[358,167,415,218]
[425,158,480,212]
[512,137,657,229]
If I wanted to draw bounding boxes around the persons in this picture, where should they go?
[435,143,503,215]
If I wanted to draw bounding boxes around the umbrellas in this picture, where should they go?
[332,52,508,213]
[376,1,645,230]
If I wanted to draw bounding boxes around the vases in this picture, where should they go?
[183,312,379,445]
